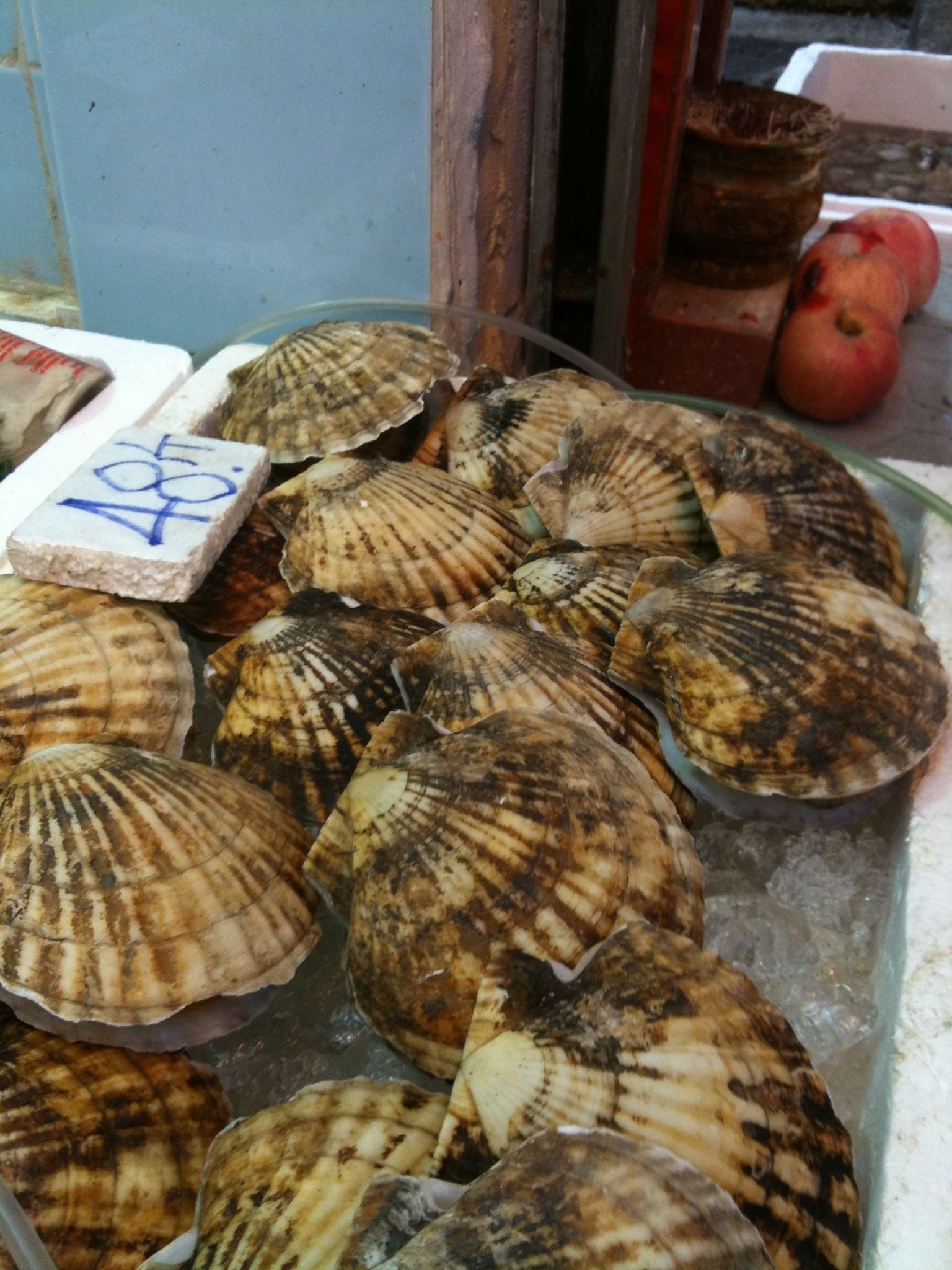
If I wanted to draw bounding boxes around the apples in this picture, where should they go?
[825,207,942,315]
[772,298,900,422]
[786,232,909,333]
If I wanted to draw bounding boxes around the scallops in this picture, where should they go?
[0,322,952,1270]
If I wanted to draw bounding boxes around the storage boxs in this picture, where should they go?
[0,318,952,1270]
[774,42,952,318]
